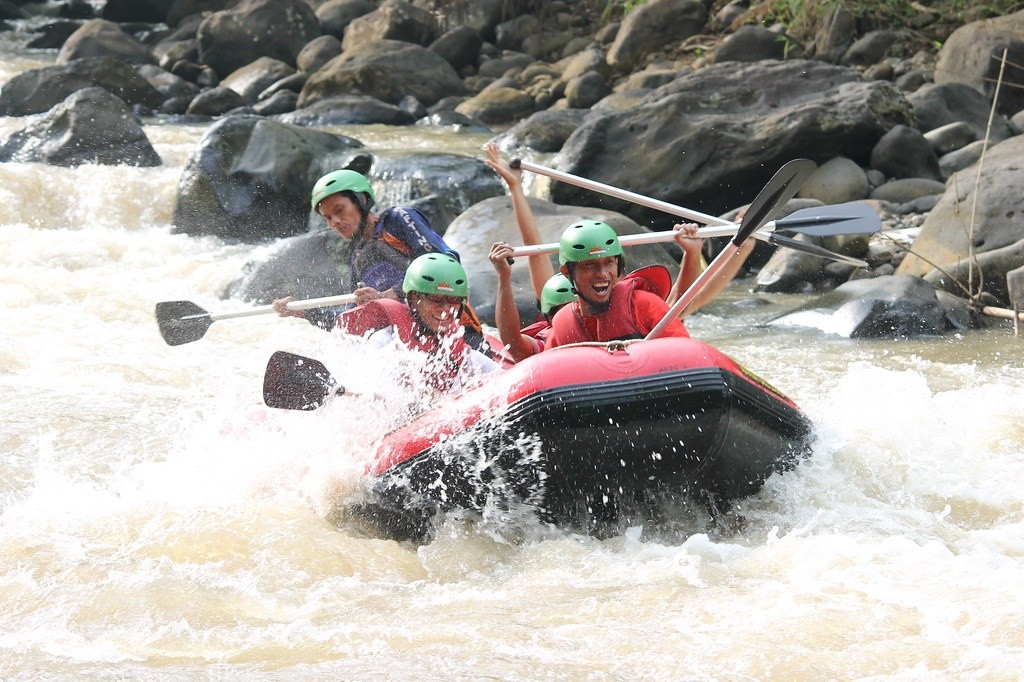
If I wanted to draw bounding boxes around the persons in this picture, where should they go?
[483,141,758,363]
[325,251,497,411]
[273,169,485,353]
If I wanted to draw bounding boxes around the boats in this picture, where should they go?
[347,318,821,551]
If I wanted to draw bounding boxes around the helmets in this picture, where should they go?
[559,220,626,266]
[311,169,376,215]
[403,253,467,297]
[540,273,579,314]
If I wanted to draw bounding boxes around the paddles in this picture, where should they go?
[261,351,438,413]
[509,200,884,260]
[155,291,364,346]
[509,156,872,270]
[648,160,820,338]
